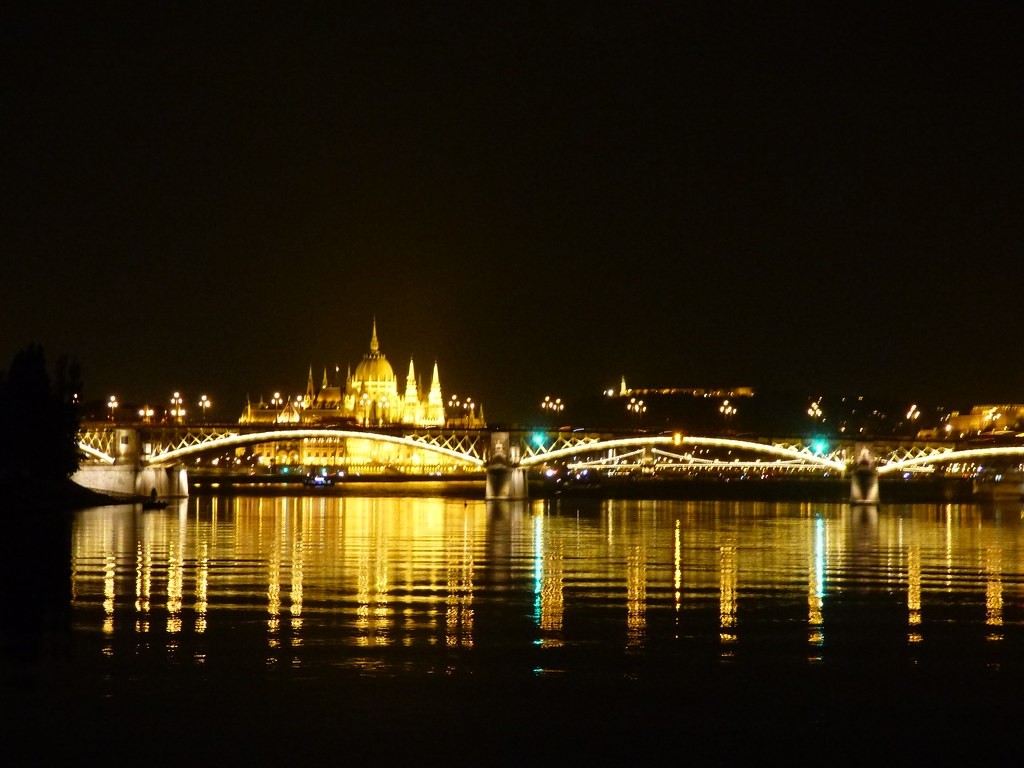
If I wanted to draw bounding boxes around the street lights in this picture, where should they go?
[271,392,283,423]
[463,397,475,429]
[542,396,564,431]
[107,395,118,423]
[906,405,920,440]
[198,395,211,420]
[138,405,155,421]
[170,392,186,426]
[807,402,823,429]
[448,394,460,430]
[720,400,737,436]
[294,395,306,422]
[627,398,647,435]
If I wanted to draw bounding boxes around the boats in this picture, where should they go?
[303,475,334,486]
[143,500,168,510]
